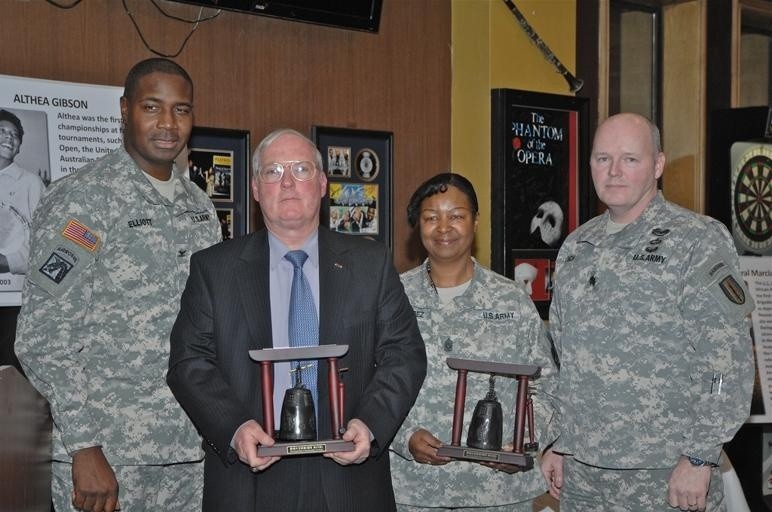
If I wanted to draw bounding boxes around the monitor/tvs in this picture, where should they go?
[169,0,383,34]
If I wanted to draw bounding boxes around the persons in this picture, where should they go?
[538,111,760,510]
[380,171,561,511]
[327,146,385,237]
[202,164,217,197]
[162,125,430,510]
[11,55,229,512]
[0,107,46,277]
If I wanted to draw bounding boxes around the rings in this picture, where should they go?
[425,459,431,466]
[249,465,258,472]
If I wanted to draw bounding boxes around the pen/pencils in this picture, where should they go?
[711,372,723,394]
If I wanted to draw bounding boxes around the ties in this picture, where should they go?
[285,249,321,438]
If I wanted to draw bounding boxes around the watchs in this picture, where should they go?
[687,454,714,467]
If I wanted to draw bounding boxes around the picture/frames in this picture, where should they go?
[313,127,394,254]
[491,88,591,321]
[188,128,250,242]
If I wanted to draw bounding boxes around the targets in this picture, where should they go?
[730,141,771,256]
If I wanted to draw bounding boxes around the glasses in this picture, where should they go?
[256,159,322,185]
